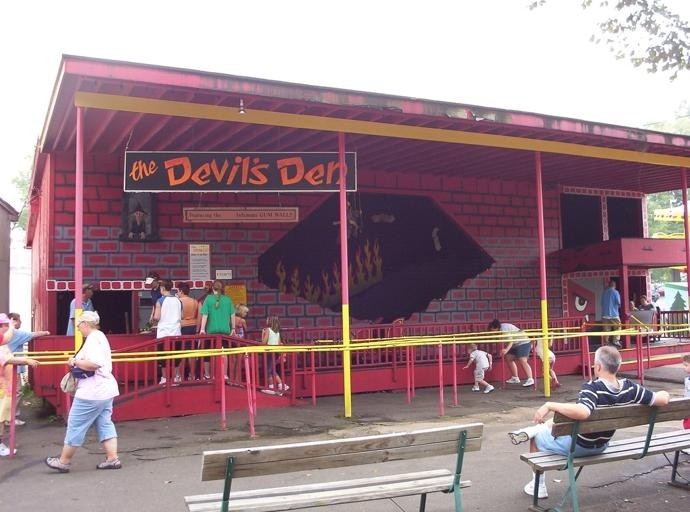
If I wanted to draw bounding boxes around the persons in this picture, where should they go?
[462,280,659,393]
[262,313,290,396]
[1,313,49,456]
[145,272,249,387]
[508,346,670,498]
[66,284,94,335]
[46,311,122,472]
[683,355,690,428]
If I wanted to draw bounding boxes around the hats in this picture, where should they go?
[0,313,13,324]
[82,283,95,291]
[144,276,158,285]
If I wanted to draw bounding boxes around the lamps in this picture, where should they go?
[238,99,247,114]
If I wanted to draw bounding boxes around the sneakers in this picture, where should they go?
[159,371,290,395]
[505,376,535,387]
[0,443,17,456]
[523,481,548,499]
[96,460,122,469]
[506,426,537,445]
[549,376,561,391]
[470,384,495,394]
[604,340,623,349]
[4,418,26,427]
[45,455,70,473]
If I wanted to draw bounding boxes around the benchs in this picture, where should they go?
[182,422,484,512]
[519,397,690,512]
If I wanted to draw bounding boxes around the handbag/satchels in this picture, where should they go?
[59,371,79,393]
[70,364,95,379]
